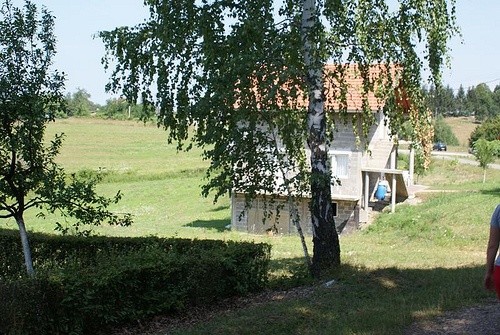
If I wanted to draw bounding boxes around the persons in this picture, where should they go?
[485,204,500,304]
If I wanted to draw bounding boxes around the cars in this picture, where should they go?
[432,144,446,151]
